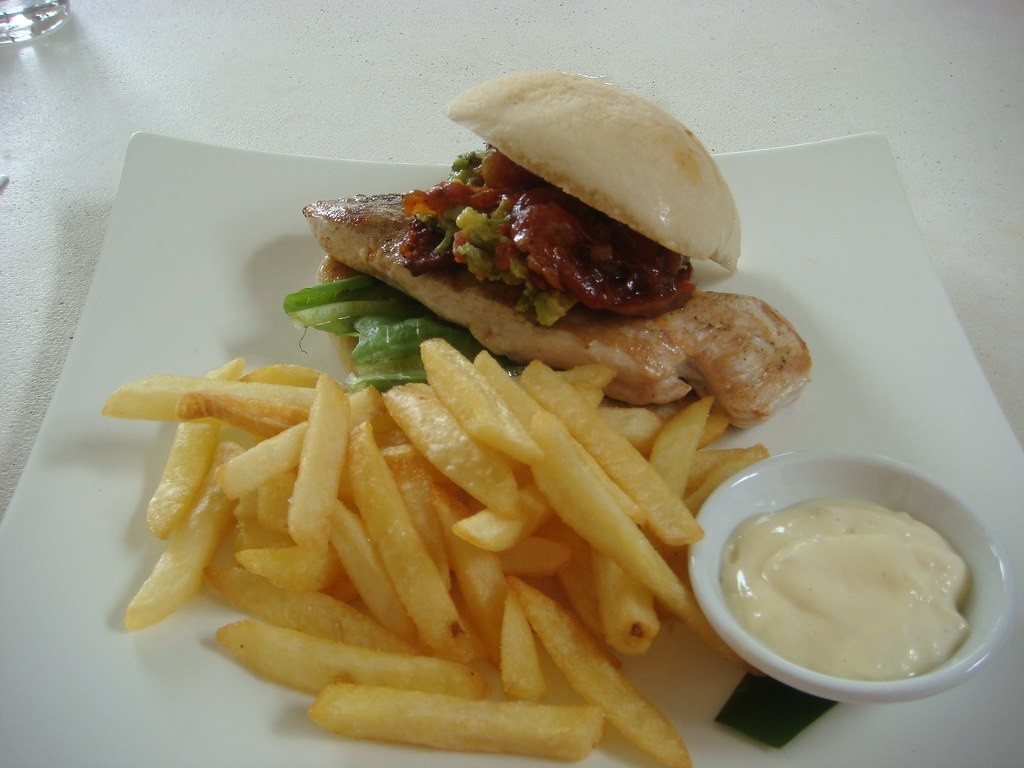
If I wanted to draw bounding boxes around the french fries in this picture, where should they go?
[100,358,695,764]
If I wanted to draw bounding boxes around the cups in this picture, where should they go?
[0,0,72,44]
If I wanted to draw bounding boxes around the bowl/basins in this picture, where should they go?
[686,444,1011,703]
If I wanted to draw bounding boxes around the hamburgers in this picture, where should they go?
[281,69,740,405]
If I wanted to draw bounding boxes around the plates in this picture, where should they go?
[0,122,1024,768]
